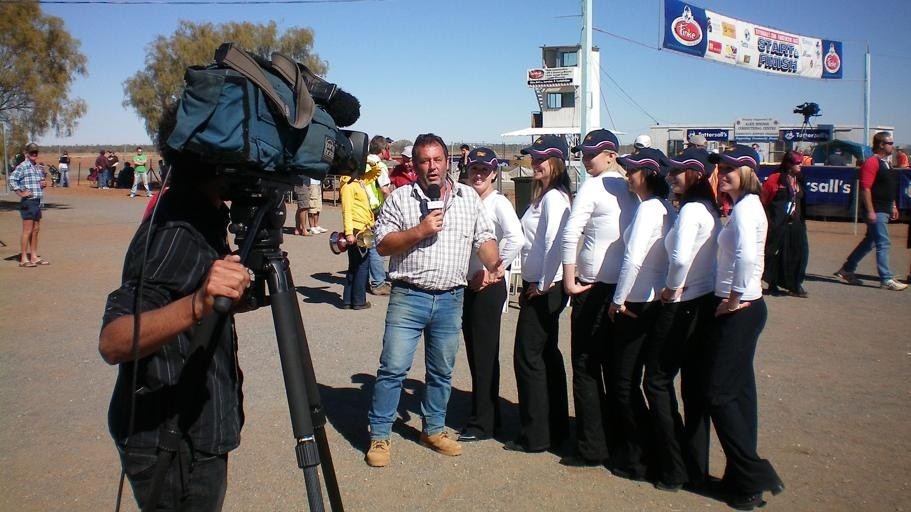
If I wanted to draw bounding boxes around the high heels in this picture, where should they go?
[727,473,785,510]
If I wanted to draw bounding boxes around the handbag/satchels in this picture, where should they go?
[764,224,790,262]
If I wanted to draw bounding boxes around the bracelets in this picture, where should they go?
[891,206,896,208]
[191,288,204,322]
[612,302,624,314]
[727,303,740,312]
[662,295,676,301]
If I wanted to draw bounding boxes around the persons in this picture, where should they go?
[760,152,809,296]
[458,148,525,442]
[824,148,847,166]
[634,134,651,155]
[8,144,153,198]
[98,99,255,512]
[643,148,723,492]
[506,136,572,452]
[892,145,908,168]
[561,129,639,466]
[837,133,908,291]
[752,144,766,162]
[684,136,718,201]
[906,171,910,284]
[607,148,677,480]
[802,149,815,165]
[366,135,505,467]
[712,140,737,154]
[7,142,51,268]
[294,136,471,309]
[702,146,785,510]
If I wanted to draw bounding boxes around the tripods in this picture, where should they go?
[143,228,343,512]
[795,121,817,152]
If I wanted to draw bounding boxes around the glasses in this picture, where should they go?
[796,161,801,165]
[752,148,755,149]
[883,142,893,145]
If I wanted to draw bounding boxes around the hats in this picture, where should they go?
[26,142,40,154]
[681,135,708,146]
[466,147,498,168]
[633,135,652,149]
[616,147,668,177]
[669,147,716,177]
[571,129,618,157]
[520,135,569,161]
[707,144,760,170]
[401,145,413,158]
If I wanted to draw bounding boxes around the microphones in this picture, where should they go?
[427,184,442,211]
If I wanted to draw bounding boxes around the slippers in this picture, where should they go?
[18,261,37,267]
[36,260,50,265]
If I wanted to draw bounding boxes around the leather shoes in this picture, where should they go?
[505,440,523,451]
[367,440,390,467]
[343,282,392,310]
[420,432,462,456]
[790,284,807,296]
[459,431,477,441]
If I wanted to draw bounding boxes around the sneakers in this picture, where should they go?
[836,269,862,285]
[879,278,907,291]
[310,226,320,234]
[315,226,327,233]
